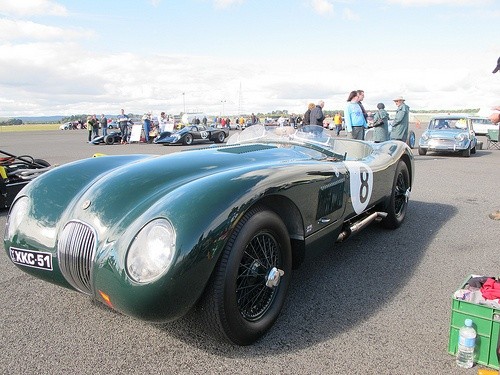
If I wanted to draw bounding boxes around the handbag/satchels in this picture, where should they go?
[373,111,384,127]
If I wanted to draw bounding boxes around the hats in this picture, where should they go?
[491,57,500,74]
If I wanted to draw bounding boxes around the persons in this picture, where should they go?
[390,96,408,142]
[310,101,326,127]
[142,112,342,143]
[344,91,372,140]
[372,103,389,143]
[302,103,316,125]
[356,89,371,123]
[85,109,134,144]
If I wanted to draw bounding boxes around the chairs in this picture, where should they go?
[333,139,374,158]
[486,129,500,150]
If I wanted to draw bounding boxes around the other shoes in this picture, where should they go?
[121,139,123,144]
[125,142,130,144]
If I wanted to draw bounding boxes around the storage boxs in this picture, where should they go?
[476,141,483,150]
[447,275,500,371]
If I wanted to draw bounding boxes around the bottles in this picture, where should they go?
[455,318,477,369]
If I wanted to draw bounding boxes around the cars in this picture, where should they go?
[258,117,375,131]
[417,116,478,159]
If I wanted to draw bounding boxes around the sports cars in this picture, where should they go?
[0,151,52,212]
[152,124,230,146]
[4,124,417,346]
[90,128,121,146]
